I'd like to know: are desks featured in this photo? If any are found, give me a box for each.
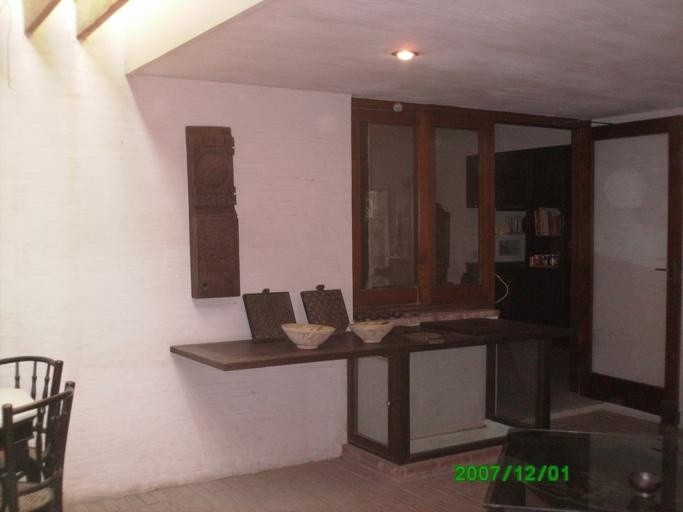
[170,318,571,464]
[484,429,683,512]
[0,388,44,482]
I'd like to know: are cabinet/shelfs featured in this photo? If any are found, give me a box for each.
[467,146,571,346]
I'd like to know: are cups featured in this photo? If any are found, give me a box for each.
[529,254,562,267]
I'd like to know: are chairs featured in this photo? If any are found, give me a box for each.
[0,381,74,512]
[0,357,62,483]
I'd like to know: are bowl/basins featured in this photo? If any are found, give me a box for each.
[348,320,394,344]
[629,470,663,498]
[280,322,336,350]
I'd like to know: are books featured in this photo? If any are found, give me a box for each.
[532,208,565,239]
